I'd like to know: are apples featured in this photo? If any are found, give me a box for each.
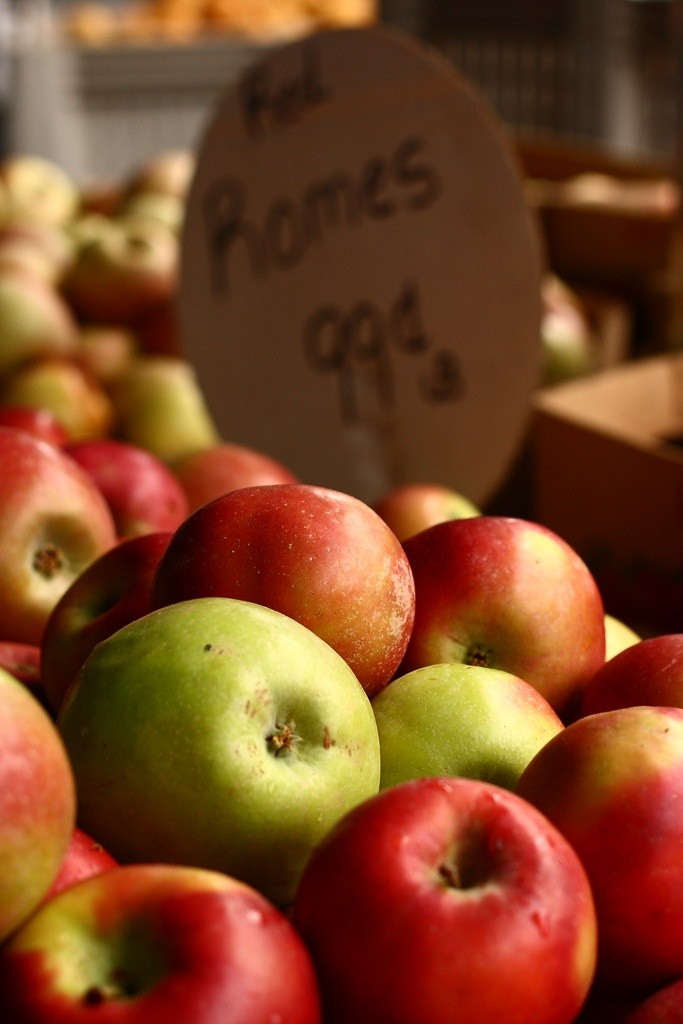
[0,138,683,1024]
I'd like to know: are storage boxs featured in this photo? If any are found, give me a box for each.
[512,121,682,347]
[531,347,683,640]
